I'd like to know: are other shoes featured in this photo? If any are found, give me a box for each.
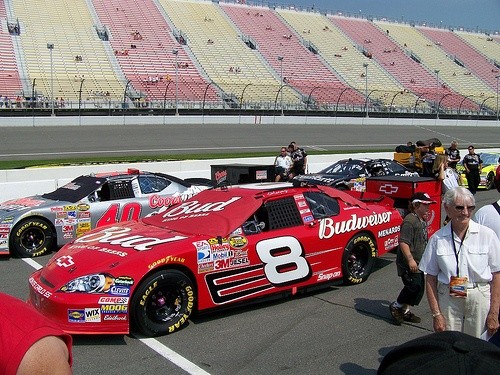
[401,306,417,322]
[389,301,403,324]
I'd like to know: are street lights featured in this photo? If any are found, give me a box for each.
[278,56,284,116]
[362,63,369,118]
[434,69,439,118]
[172,49,180,115]
[47,42,56,115]
[495,74,500,121]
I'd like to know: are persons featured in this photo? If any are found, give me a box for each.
[1,291,76,375]
[59,86,64,92]
[0,0,500,121]
[419,146,439,177]
[418,180,500,342]
[493,157,500,191]
[445,139,461,170]
[274,141,309,183]
[389,191,437,326]
[462,145,484,194]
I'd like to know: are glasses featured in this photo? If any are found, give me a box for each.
[453,204,476,211]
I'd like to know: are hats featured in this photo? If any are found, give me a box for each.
[410,192,437,205]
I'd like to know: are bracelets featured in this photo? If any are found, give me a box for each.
[432,312,442,318]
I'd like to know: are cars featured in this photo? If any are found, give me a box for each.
[27,179,403,339]
[456,152,500,190]
[0,168,213,260]
[288,157,420,189]
